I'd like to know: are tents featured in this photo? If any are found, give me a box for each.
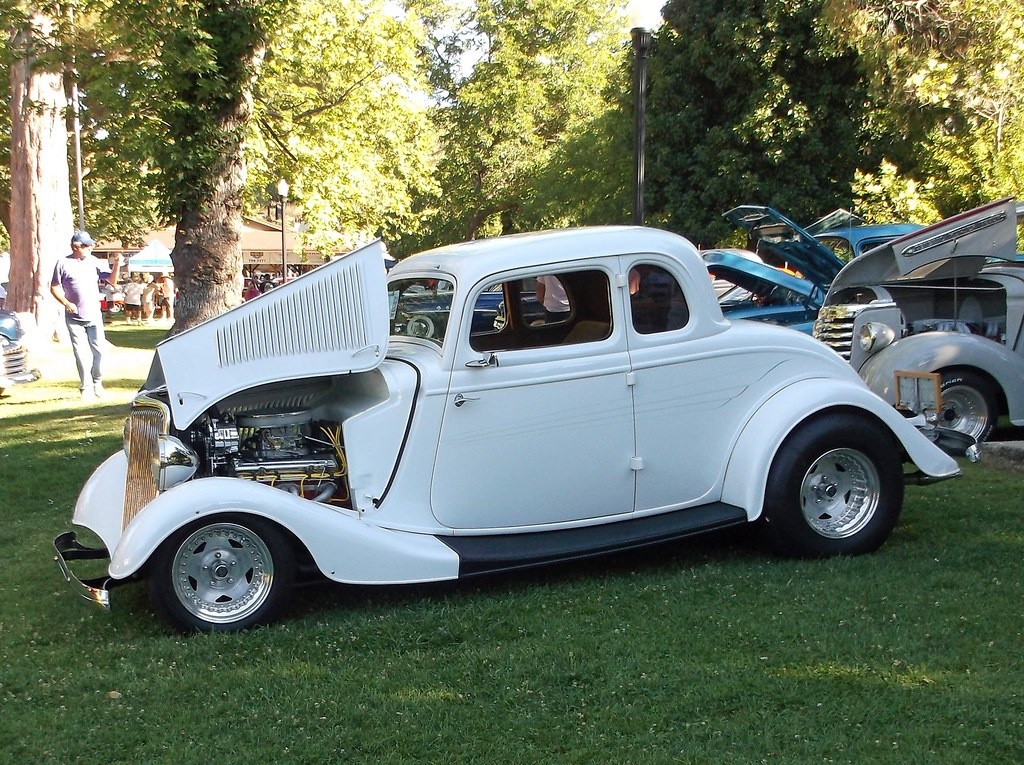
[126,238,177,282]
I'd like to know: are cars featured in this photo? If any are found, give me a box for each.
[50,222,982,635]
[696,194,1024,457]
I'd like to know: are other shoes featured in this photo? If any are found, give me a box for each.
[126,317,175,325]
[80,389,96,402]
[94,380,104,398]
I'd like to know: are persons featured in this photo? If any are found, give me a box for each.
[0,284,7,311]
[536,272,572,326]
[95,268,177,326]
[51,230,125,401]
[237,271,299,303]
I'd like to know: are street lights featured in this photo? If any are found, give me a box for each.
[277,178,291,283]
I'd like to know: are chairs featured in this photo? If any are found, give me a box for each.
[561,318,609,344]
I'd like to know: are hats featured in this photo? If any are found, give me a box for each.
[160,272,172,279]
[70,231,97,246]
